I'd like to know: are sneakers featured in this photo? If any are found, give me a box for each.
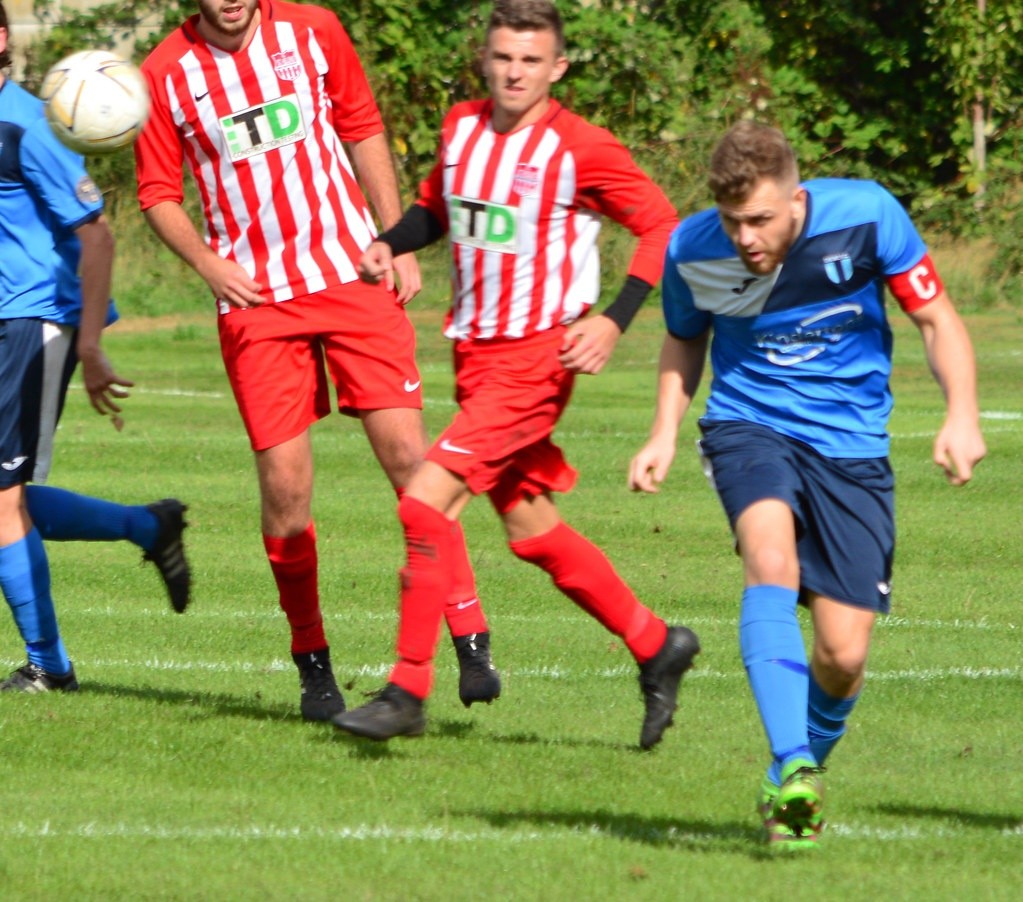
[637,624,700,748]
[0,662,79,695]
[774,757,826,833]
[450,631,500,709]
[140,499,193,613]
[759,777,822,852]
[331,683,426,739]
[292,648,347,721]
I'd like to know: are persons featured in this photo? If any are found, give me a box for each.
[332,0,701,751]
[627,122,987,858]
[133,0,502,727]
[0,0,194,693]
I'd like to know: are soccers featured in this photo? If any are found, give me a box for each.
[40,48,149,155]
